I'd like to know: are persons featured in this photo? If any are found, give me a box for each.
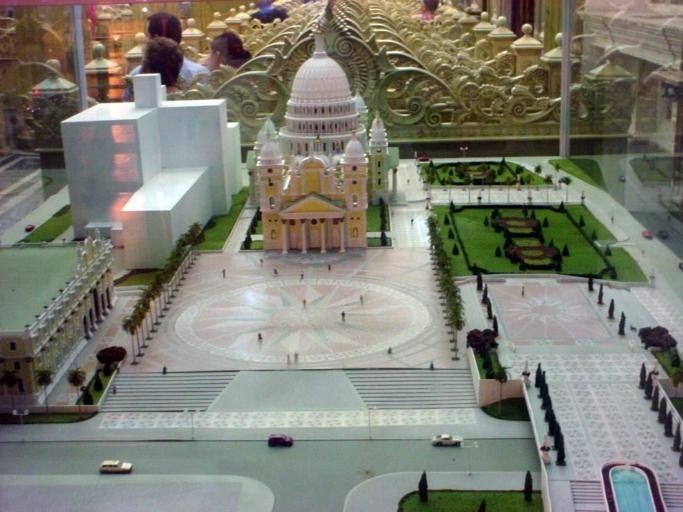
[408,0,439,21]
[126,35,185,102]
[120,10,212,102]
[248,0,288,29]
[206,28,251,71]
